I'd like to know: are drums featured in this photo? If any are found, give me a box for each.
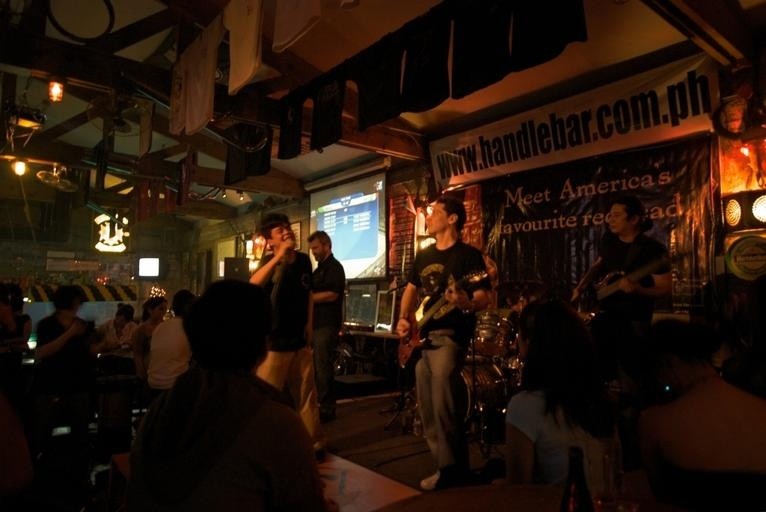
[474,312,512,358]
[501,365,522,391]
[450,362,507,432]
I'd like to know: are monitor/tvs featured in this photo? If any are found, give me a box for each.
[138,257,165,280]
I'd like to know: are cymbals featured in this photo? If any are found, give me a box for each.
[493,282,546,298]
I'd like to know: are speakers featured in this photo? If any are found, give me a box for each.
[224,257,249,282]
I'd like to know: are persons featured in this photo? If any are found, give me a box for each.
[638,326,765,469]
[505,301,585,485]
[397,195,493,491]
[569,198,673,333]
[0,212,348,511]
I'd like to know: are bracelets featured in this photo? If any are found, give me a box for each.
[394,312,410,322]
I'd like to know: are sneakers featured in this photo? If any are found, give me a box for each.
[420,470,440,491]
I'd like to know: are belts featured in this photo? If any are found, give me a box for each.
[429,329,455,337]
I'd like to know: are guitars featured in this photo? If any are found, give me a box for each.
[398,270,488,368]
[570,245,693,320]
